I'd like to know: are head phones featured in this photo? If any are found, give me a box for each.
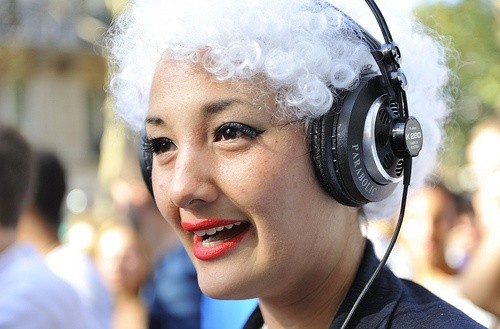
[137,0,422,207]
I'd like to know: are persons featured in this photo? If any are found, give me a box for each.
[0,117,258,329]
[95,0,490,329]
[352,104,499,329]
